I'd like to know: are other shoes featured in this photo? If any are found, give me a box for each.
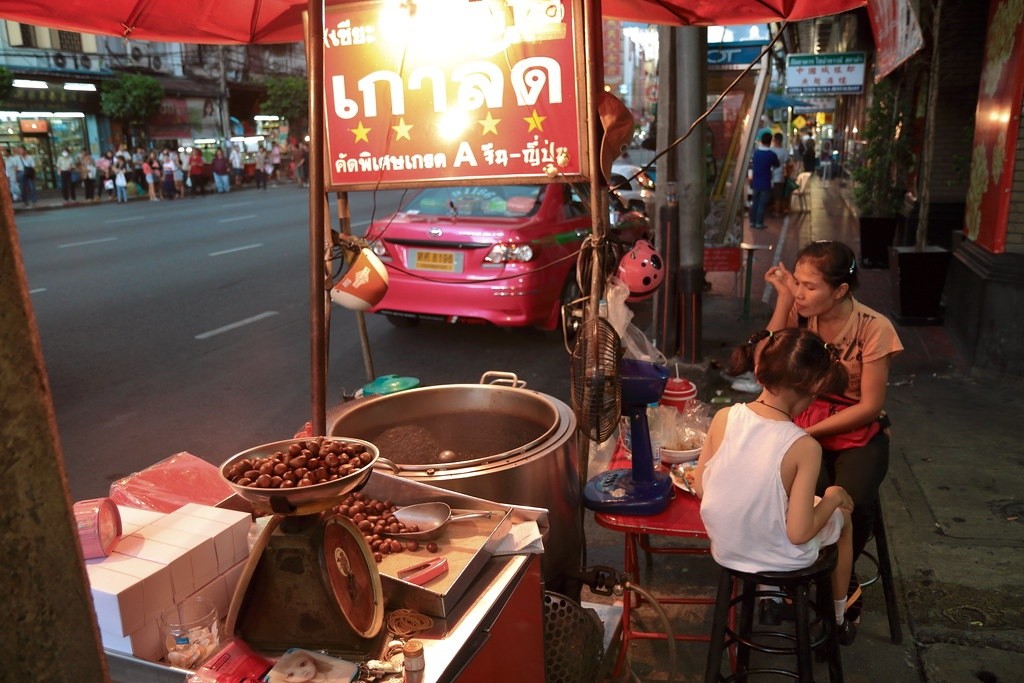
[750,223,768,229]
[830,617,857,644]
[758,599,790,625]
[149,197,160,201]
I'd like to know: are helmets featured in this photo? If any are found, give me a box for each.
[614,240,665,303]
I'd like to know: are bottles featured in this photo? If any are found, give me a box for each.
[646,403,662,468]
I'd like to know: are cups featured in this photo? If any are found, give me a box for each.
[160,597,218,669]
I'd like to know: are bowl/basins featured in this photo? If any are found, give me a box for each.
[651,428,706,463]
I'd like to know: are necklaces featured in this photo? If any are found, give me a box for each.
[755,400,793,421]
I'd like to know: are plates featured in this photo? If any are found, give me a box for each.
[668,461,699,495]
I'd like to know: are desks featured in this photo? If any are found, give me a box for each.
[595,421,746,682]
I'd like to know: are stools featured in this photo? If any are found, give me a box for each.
[845,488,903,645]
[705,547,839,683]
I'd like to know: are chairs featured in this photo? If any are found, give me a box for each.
[787,170,813,215]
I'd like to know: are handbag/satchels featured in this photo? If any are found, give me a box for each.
[104,179,115,190]
[24,166,36,179]
[793,390,890,449]
[186,176,193,187]
[115,169,128,187]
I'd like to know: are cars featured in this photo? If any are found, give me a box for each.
[361,182,654,343]
[533,164,655,227]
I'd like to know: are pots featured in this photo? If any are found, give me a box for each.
[329,369,559,471]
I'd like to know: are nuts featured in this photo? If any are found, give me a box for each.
[330,493,438,562]
[232,435,373,487]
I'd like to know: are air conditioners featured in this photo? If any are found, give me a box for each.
[46,40,172,72]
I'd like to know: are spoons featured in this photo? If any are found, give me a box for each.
[670,464,695,496]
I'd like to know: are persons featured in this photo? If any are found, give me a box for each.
[770,131,816,218]
[754,239,905,624]
[750,132,780,229]
[3,138,310,208]
[199,99,220,140]
[695,327,869,647]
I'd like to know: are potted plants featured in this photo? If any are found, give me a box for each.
[884,1,965,327]
[852,80,911,270]
